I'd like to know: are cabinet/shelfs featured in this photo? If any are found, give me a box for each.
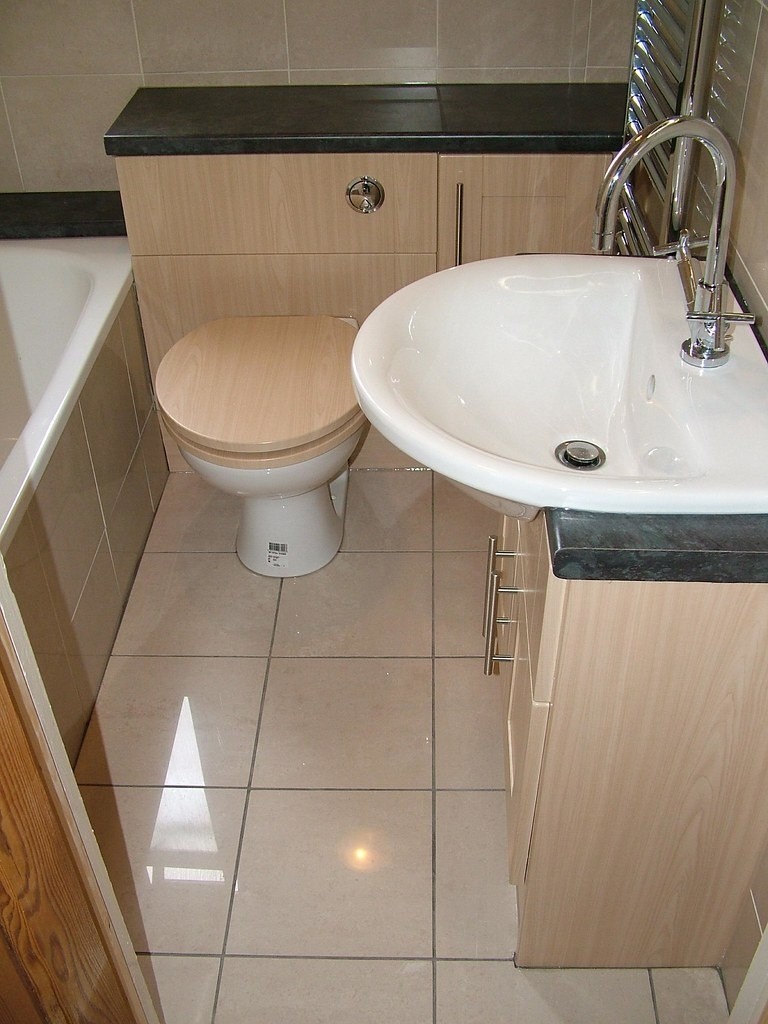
[100,79,675,474]
[482,505,768,972]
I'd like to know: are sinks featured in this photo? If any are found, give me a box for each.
[349,253,768,516]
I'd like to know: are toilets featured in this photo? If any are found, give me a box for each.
[154,314,369,579]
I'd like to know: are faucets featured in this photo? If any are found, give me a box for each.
[591,115,756,369]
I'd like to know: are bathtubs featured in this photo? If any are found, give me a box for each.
[0,236,171,774]
